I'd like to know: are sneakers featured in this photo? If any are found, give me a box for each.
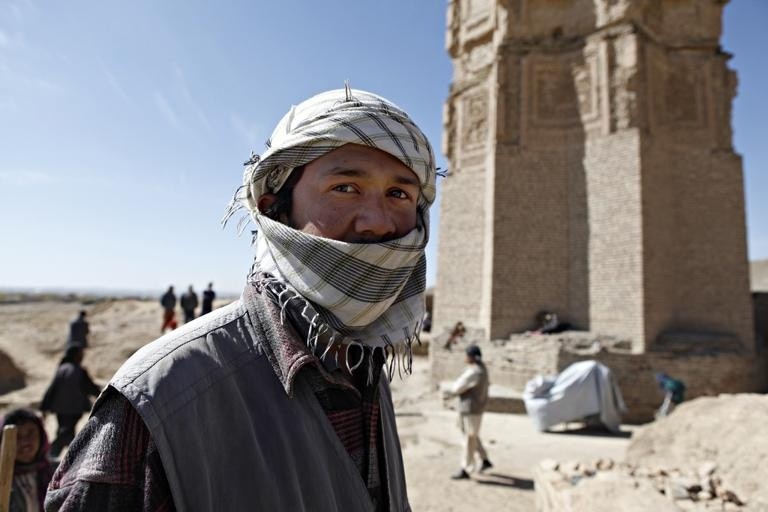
[449,460,492,481]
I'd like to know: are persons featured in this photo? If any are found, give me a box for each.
[42,87,439,512]
[160,285,178,334]
[655,373,686,416]
[436,345,494,481]
[181,284,199,324]
[0,407,54,512]
[200,282,216,316]
[67,309,90,350]
[38,342,102,458]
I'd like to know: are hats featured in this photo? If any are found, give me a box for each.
[466,345,481,358]
